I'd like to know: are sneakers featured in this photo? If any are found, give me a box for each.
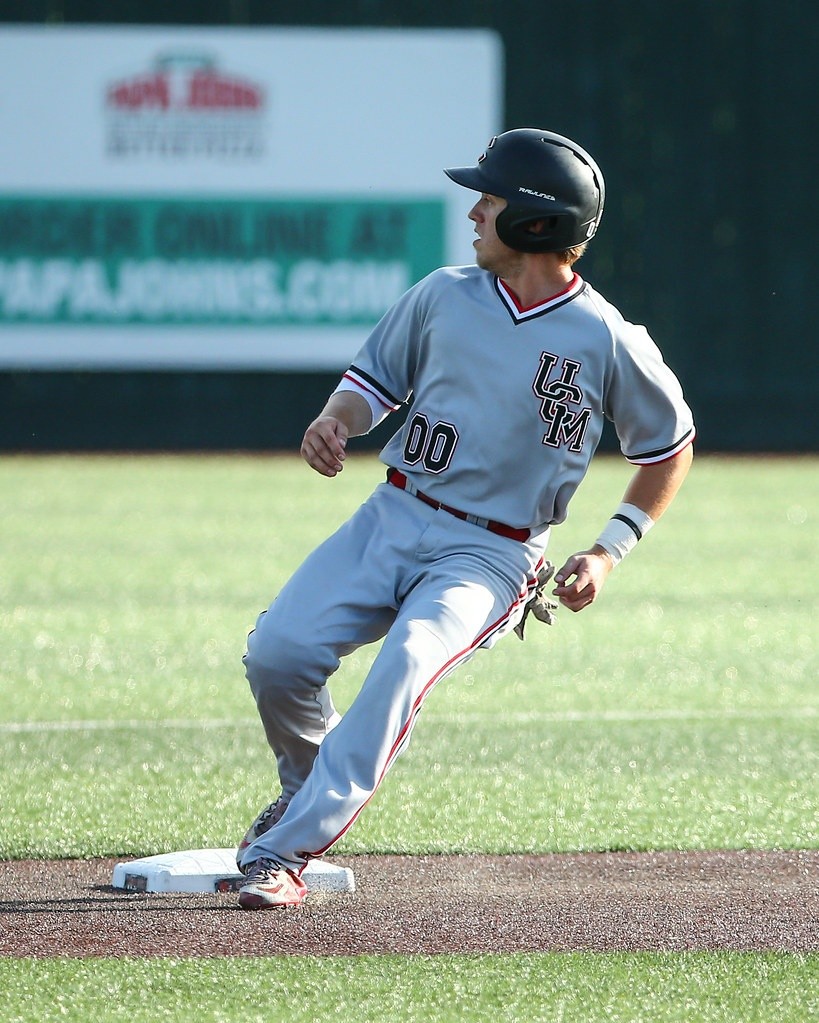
[237,855,307,909]
[235,794,292,873]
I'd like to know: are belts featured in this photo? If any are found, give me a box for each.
[387,469,530,544]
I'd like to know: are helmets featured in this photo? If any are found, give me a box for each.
[443,128,606,253]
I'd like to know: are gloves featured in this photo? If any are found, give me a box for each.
[513,559,559,641]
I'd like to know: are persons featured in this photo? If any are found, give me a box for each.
[236,129,696,910]
